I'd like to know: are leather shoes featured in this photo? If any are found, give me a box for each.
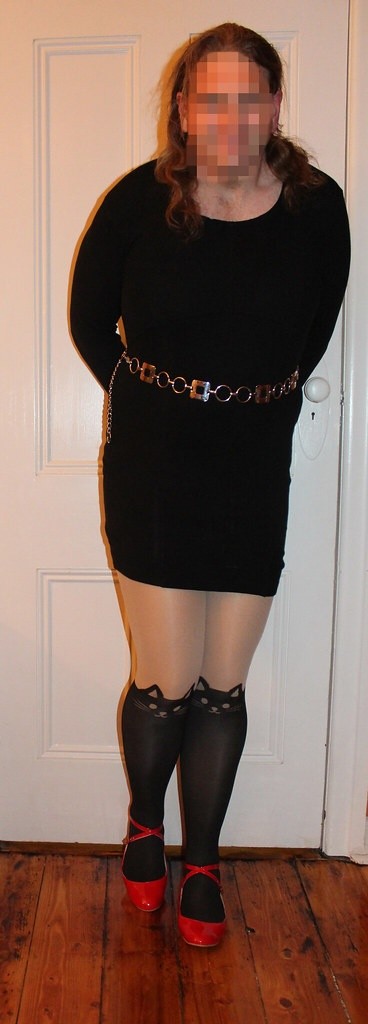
[177,860,228,947]
[121,806,169,912]
